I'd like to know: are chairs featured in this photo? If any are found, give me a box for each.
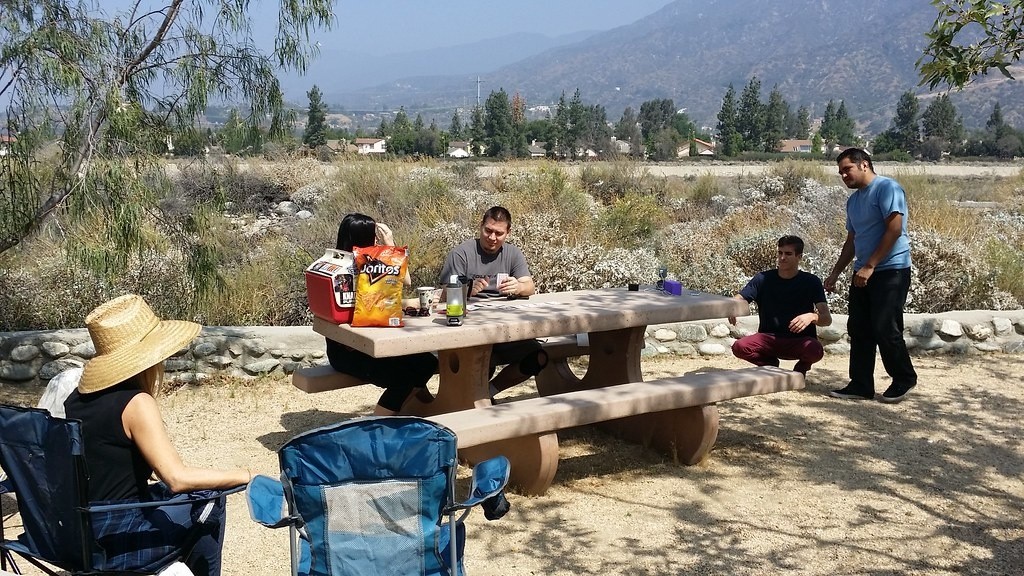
[246,415,508,576]
[0,403,249,576]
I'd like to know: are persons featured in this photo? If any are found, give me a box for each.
[325,214,440,418]
[63,294,263,576]
[732,236,833,380]
[825,148,917,402]
[440,208,550,407]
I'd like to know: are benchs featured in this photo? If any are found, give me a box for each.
[291,339,805,495]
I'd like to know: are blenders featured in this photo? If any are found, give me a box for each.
[444,283,464,325]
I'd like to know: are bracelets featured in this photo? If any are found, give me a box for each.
[246,468,252,481]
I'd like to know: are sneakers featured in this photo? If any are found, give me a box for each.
[882,379,917,402]
[830,383,865,399]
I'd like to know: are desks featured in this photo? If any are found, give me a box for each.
[314,286,748,495]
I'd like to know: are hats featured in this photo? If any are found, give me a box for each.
[78,294,202,394]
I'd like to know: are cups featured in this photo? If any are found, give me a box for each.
[416,287,434,316]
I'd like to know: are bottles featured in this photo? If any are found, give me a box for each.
[455,275,473,316]
